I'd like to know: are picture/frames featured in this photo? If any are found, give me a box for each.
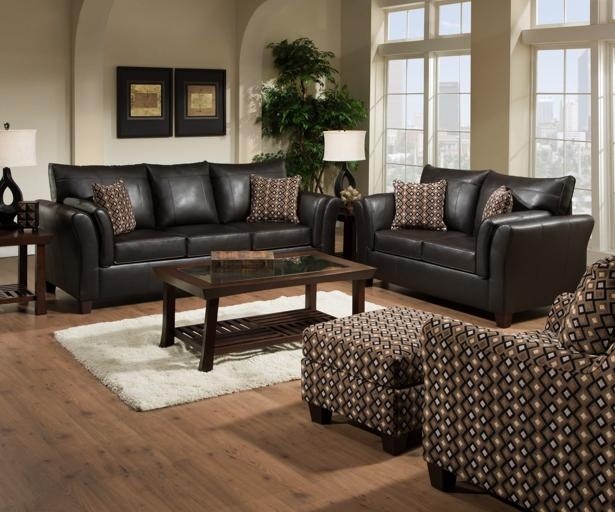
[174,67,227,137]
[116,64,173,138]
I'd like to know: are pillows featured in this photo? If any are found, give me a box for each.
[558,259,614,352]
[473,168,576,230]
[89,178,138,235]
[147,161,219,228]
[209,158,286,224]
[481,184,513,220]
[422,163,487,234]
[49,162,156,232]
[248,172,302,226]
[390,177,447,232]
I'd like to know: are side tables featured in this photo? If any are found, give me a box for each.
[1,227,52,315]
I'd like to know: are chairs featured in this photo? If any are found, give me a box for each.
[421,255,615,511]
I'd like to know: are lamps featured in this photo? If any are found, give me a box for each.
[1,120,37,232]
[322,128,367,197]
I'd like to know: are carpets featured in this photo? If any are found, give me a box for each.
[51,289,384,413]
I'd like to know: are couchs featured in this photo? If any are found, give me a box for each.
[356,164,597,327]
[34,160,340,316]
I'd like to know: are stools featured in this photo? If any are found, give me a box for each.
[301,303,479,456]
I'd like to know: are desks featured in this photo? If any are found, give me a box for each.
[337,213,357,258]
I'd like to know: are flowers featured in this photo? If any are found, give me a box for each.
[339,185,360,204]
